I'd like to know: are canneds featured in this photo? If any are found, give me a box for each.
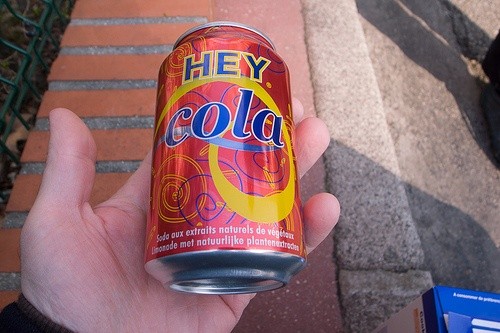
[144,19,306,296]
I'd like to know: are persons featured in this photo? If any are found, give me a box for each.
[1,97,339,332]
